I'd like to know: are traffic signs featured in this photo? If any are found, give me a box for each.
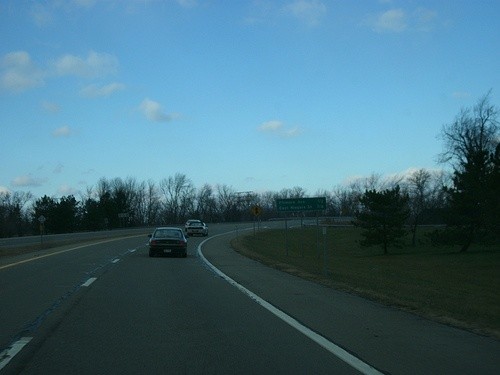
[276,197,327,212]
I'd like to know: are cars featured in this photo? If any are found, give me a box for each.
[147,227,188,258]
[185,219,208,237]
